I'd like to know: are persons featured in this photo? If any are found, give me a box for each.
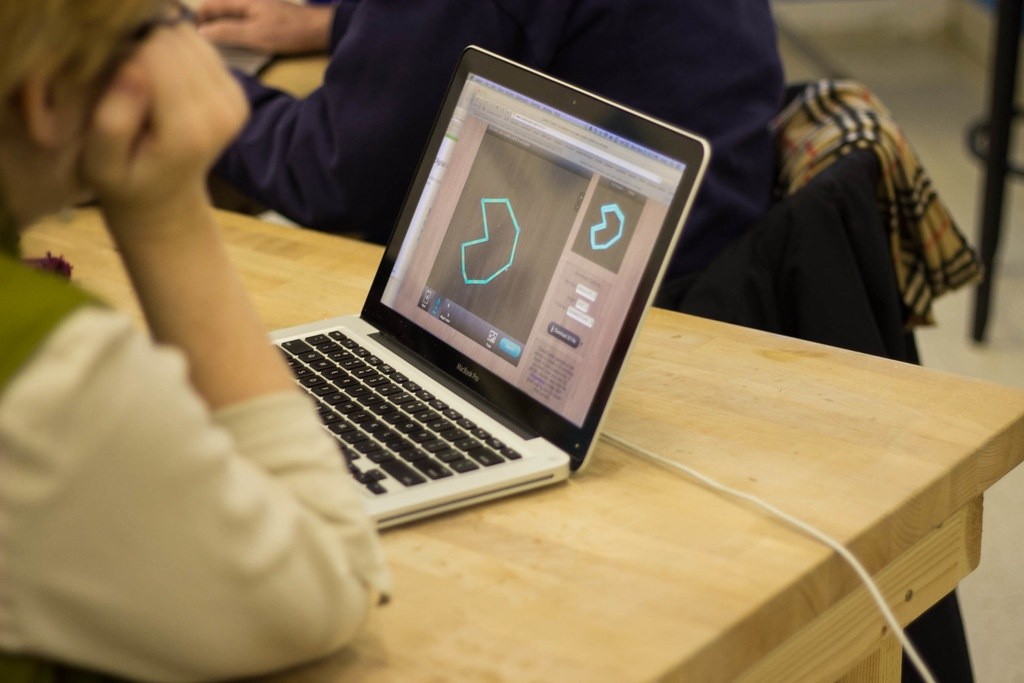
[0,0,393,683]
[196,0,784,313]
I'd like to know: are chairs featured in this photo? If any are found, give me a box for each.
[677,80,979,682]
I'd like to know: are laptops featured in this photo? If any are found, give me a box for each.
[265,43,711,528]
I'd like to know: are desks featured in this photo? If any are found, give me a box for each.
[25,206,1024,682]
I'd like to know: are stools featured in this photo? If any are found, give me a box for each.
[964,1,1023,341]
